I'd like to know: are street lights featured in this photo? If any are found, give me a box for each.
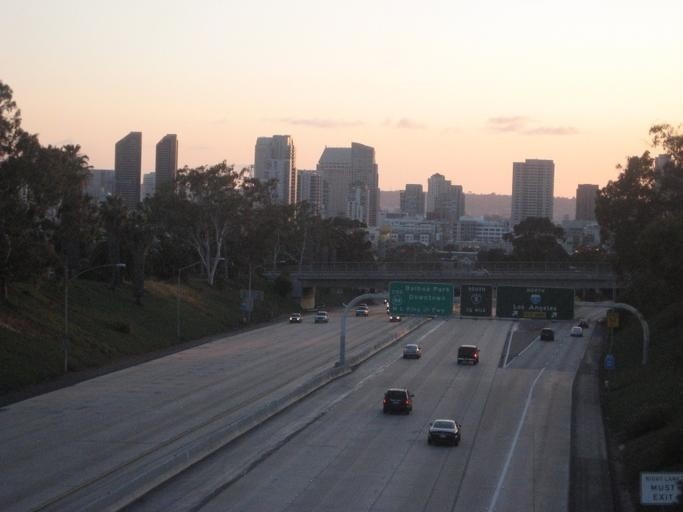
[176,257,225,346]
[64,264,127,375]
[247,260,287,324]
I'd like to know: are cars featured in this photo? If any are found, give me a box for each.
[356,303,369,316]
[403,343,423,359]
[571,319,589,337]
[383,388,415,414]
[289,312,303,323]
[390,315,401,322]
[541,328,555,341]
[428,419,462,446]
[314,311,330,323]
[457,345,481,365]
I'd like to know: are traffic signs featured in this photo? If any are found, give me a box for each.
[496,285,574,319]
